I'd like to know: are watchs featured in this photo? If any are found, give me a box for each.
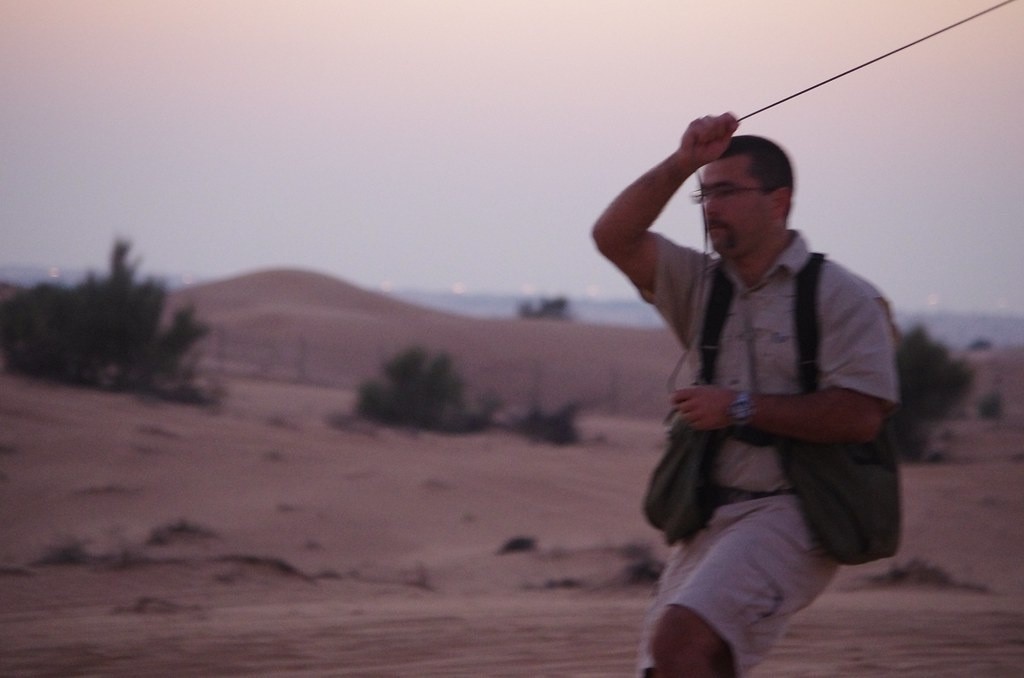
[729,390,755,426]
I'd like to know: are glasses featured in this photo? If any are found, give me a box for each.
[689,183,773,206]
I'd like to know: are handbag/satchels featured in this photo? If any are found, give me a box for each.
[781,430,900,566]
[640,407,726,545]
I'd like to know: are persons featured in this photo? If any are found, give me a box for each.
[593,113,904,678]
